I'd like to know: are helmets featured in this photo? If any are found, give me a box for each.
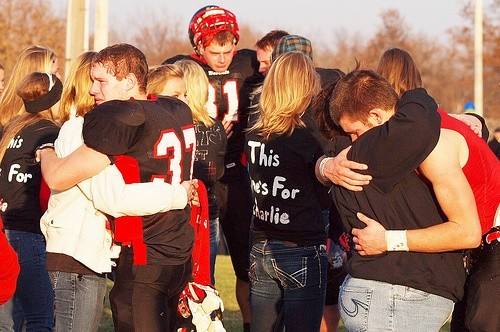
[188,4,240,56]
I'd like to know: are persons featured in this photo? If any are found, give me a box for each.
[315,69,500,332]
[0,0,500,332]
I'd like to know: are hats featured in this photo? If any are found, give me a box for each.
[271,34,313,63]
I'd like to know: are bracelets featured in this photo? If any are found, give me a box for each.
[386,230,408,251]
[319,157,333,182]
